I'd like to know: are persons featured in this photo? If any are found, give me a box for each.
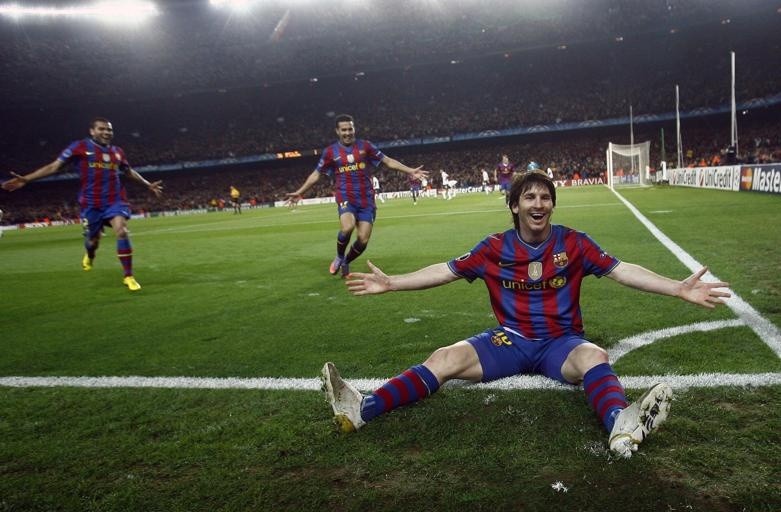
[0,1,781,230]
[1,116,167,293]
[283,114,431,281]
[320,167,736,461]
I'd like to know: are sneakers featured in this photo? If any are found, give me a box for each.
[83,251,94,271]
[341,256,350,277]
[607,381,680,461]
[329,255,343,275]
[321,359,368,436]
[123,275,142,291]
